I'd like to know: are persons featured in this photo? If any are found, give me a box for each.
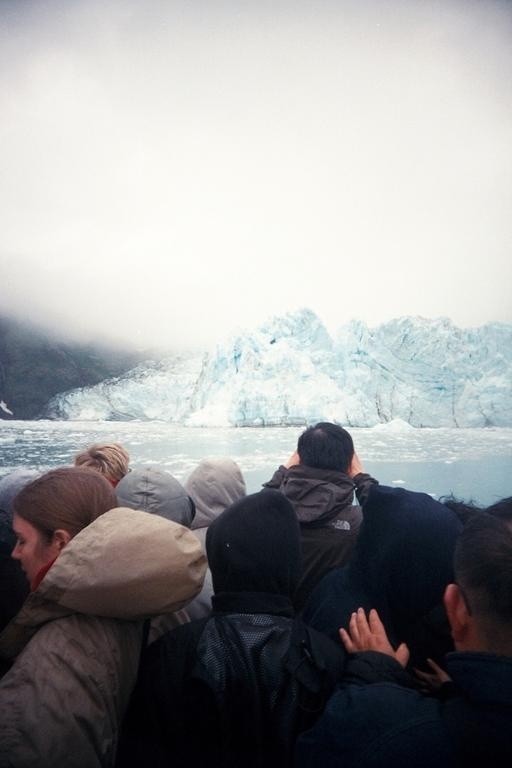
[1,421,512,767]
